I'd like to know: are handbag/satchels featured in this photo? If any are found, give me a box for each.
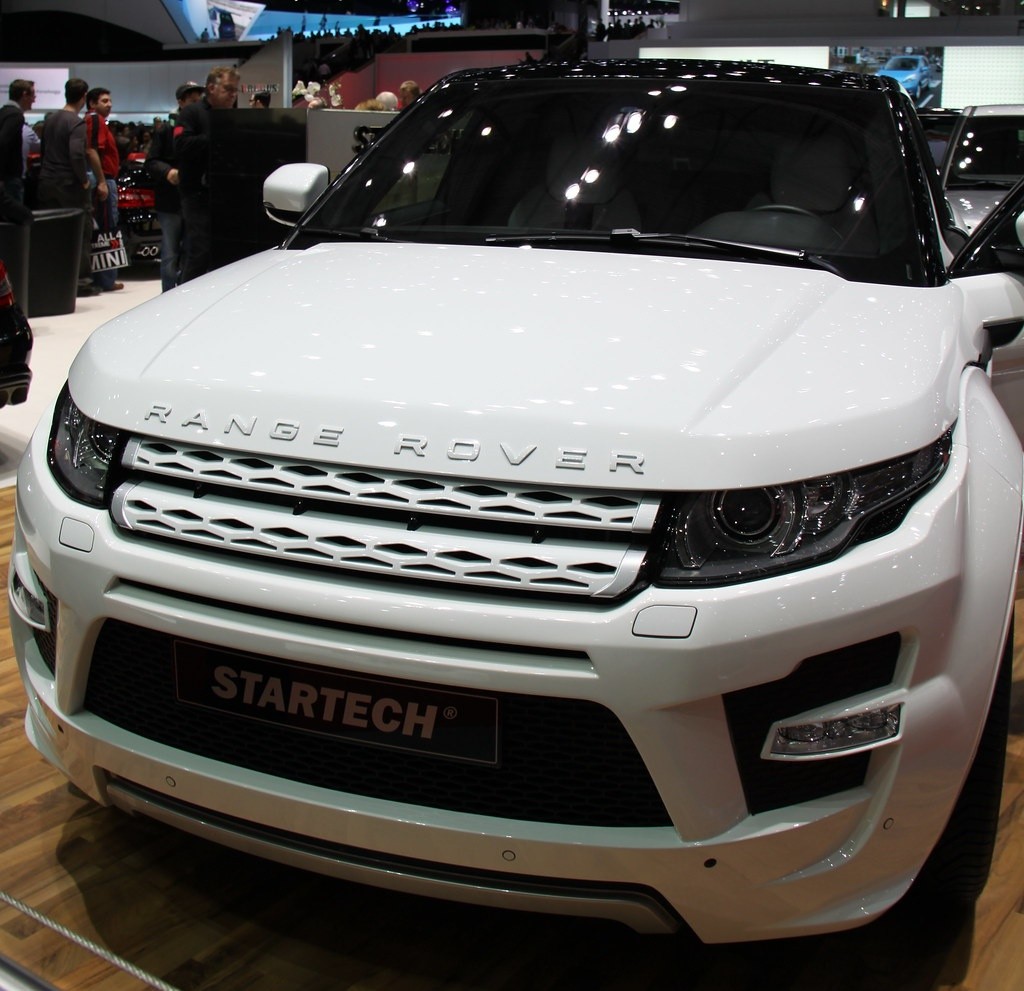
[91,227,132,271]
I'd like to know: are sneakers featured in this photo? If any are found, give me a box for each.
[102,283,124,291]
[77,286,101,296]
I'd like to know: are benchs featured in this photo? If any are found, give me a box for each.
[605,108,830,236]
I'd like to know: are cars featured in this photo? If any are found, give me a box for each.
[112,154,167,262]
[10,64,1023,977]
[876,55,932,101]
[936,105,1024,253]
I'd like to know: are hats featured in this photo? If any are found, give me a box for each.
[176,85,205,98]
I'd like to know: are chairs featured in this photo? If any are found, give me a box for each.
[743,132,881,254]
[970,120,1024,174]
[508,129,644,232]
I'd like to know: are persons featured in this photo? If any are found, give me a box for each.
[0,80,53,210]
[39,77,93,273]
[82,88,123,292]
[354,90,398,111]
[399,80,421,110]
[143,81,207,294]
[249,90,272,109]
[107,117,166,170]
[172,69,240,285]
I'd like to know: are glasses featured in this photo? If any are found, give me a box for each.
[249,99,255,102]
